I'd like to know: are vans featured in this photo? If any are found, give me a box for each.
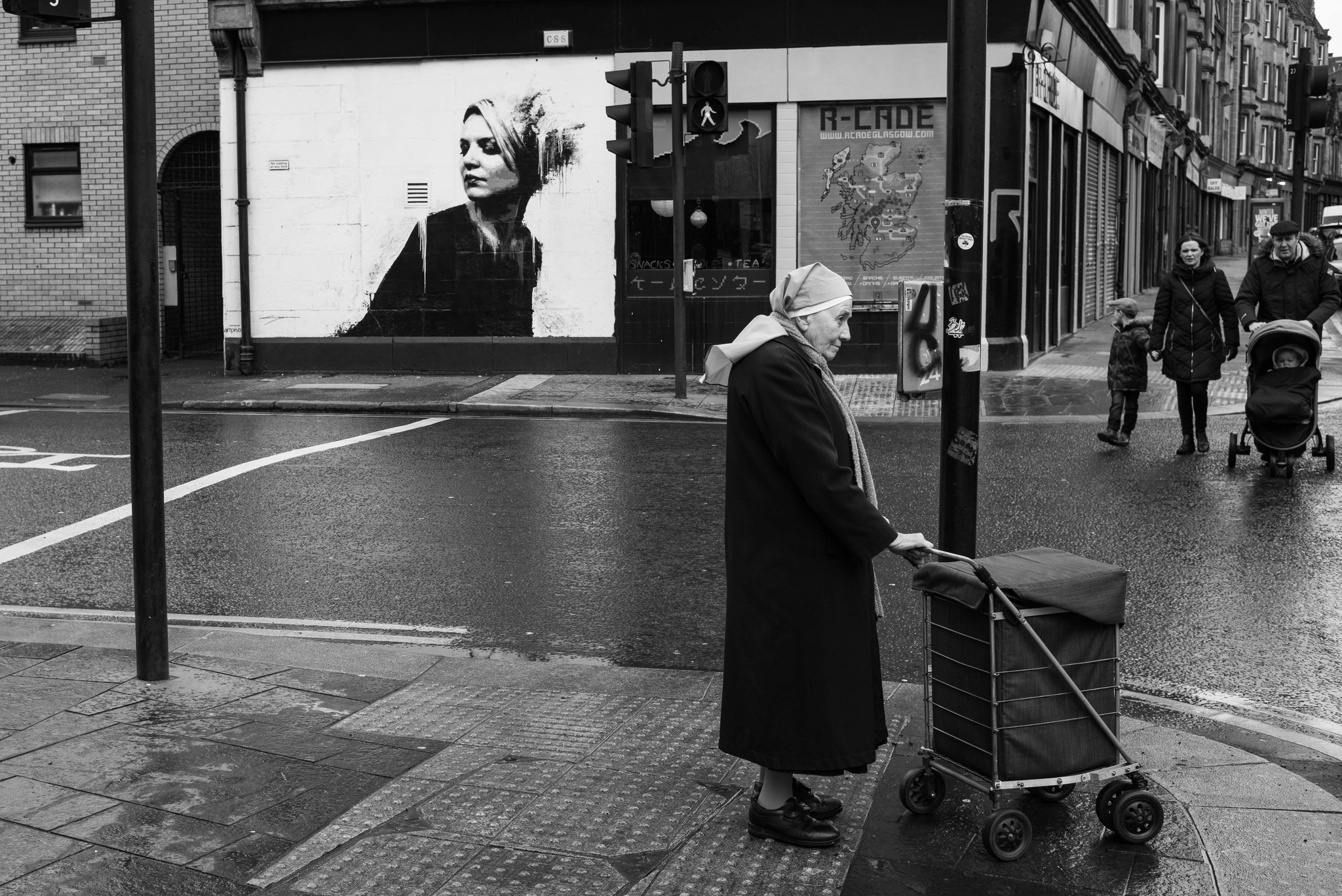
[1322,205,1342,225]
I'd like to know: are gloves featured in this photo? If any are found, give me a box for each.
[1150,348,1164,362]
[1225,344,1238,361]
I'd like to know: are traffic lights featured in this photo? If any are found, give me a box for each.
[1285,63,1331,132]
[2,0,80,23]
[605,61,655,169]
[686,60,729,135]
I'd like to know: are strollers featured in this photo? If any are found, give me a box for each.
[1227,319,1335,479]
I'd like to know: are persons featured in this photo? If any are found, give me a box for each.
[699,262,934,848]
[1149,232,1240,454]
[1234,221,1342,462]
[1272,343,1308,369]
[349,95,573,336]
[1097,297,1161,446]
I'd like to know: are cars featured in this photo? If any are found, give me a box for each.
[1320,222,1342,291]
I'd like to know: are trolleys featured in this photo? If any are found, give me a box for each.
[898,540,1166,864]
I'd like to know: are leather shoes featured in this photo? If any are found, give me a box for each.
[751,778,842,821]
[748,793,841,848]
[1097,428,1119,446]
[1195,431,1209,453]
[1176,433,1196,455]
[1111,433,1131,447]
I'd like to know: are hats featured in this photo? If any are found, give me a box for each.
[1109,297,1139,315]
[1269,220,1300,236]
